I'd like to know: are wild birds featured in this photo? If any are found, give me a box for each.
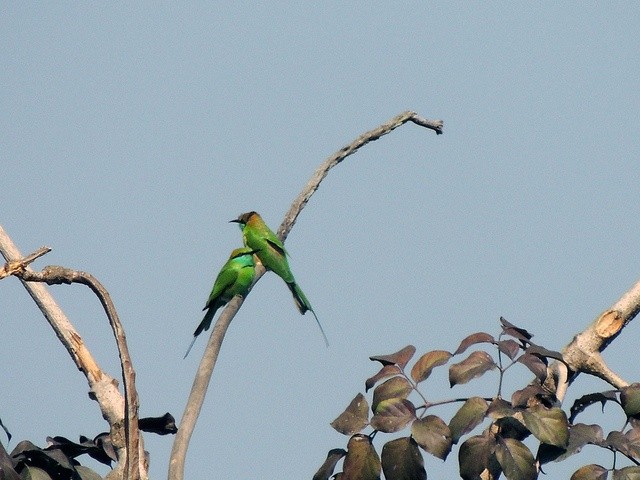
[181,245,260,360]
[230,210,330,346]
